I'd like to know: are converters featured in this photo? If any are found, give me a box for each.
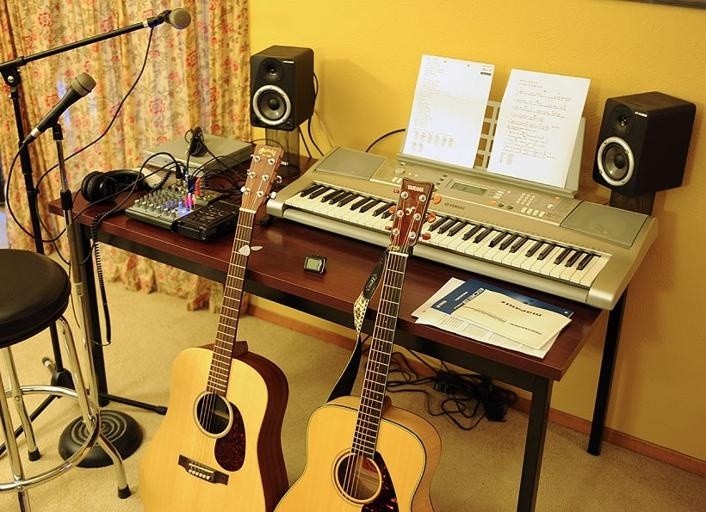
[434,370,462,395]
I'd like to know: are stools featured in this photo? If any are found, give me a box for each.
[0,250,131,512]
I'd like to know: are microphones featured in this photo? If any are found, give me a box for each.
[22,72,96,146]
[164,8,191,29]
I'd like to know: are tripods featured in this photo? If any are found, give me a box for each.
[0,11,167,456]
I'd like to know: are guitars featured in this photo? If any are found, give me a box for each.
[274,178,442,512]
[138,143,289,511]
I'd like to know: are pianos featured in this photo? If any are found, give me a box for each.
[265,147,659,312]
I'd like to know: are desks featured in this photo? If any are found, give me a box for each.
[51,155,647,510]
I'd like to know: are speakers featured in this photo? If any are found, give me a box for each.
[250,46,313,131]
[592,92,696,197]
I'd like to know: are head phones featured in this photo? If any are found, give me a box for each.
[81,170,144,203]
